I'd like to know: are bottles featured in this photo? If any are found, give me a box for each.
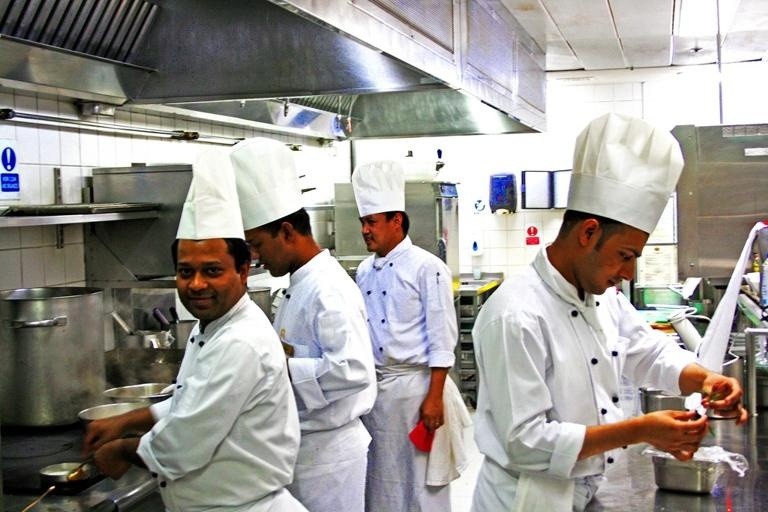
[753,253,768,307]
[169,305,180,322]
[107,309,134,336]
[152,307,171,333]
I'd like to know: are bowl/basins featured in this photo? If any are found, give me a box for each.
[105,382,172,402]
[79,400,153,424]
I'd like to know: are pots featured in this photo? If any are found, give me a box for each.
[38,450,104,490]
[1,282,105,432]
[638,386,685,415]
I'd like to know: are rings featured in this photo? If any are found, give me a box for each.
[435,422,440,425]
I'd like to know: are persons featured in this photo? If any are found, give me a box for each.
[469,209,750,512]
[355,209,459,512]
[243,208,378,511]
[80,238,311,511]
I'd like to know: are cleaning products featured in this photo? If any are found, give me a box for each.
[471,254,482,280]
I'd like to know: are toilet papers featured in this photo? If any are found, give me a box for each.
[669,311,702,351]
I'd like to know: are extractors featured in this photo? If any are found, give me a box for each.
[0,1,168,74]
[272,95,361,120]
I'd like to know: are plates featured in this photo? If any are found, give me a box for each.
[731,332,759,347]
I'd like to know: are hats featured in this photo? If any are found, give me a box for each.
[175,155,247,241]
[567,112,685,234]
[351,159,405,218]
[230,137,303,231]
